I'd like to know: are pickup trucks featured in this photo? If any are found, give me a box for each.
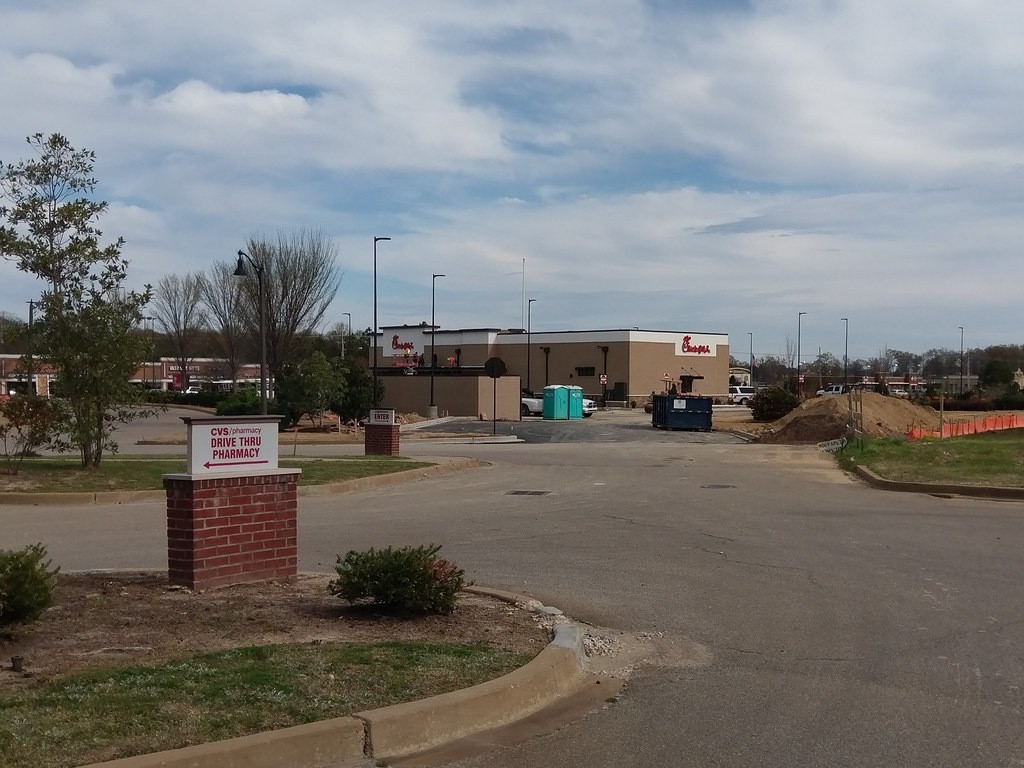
[521,397,598,418]
[816,385,845,397]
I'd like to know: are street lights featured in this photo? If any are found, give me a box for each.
[798,312,806,398]
[373,236,391,408]
[959,327,964,394]
[343,313,351,335]
[141,316,156,389]
[748,333,753,385]
[231,249,268,415]
[840,318,847,391]
[528,298,537,393]
[431,274,445,405]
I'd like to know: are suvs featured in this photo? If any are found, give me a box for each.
[890,390,908,395]
[729,386,756,405]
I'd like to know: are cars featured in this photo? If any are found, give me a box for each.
[256,386,274,398]
[182,386,202,393]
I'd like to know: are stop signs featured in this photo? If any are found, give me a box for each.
[600,375,607,384]
[665,373,669,380]
